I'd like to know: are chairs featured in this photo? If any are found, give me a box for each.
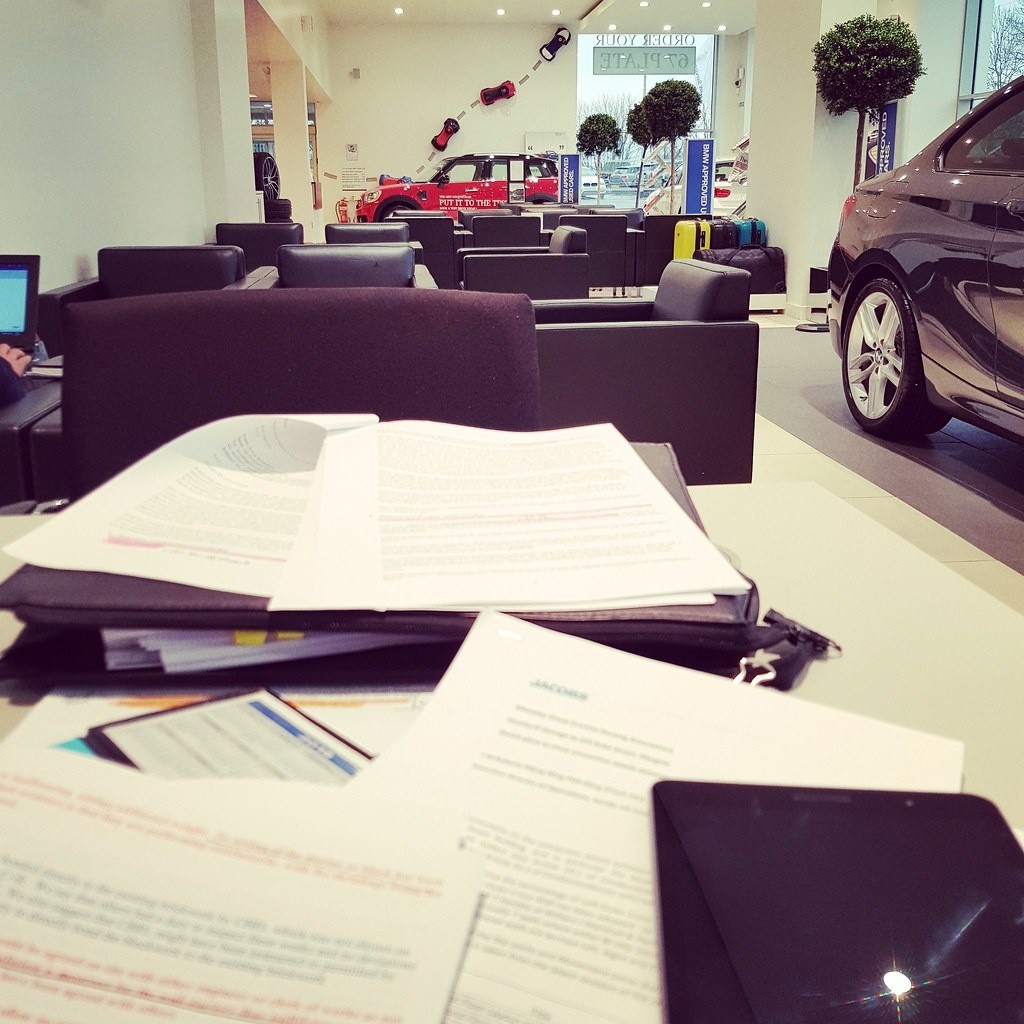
[0,202,759,486]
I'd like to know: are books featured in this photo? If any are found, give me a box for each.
[25,352,64,380]
[55,690,378,794]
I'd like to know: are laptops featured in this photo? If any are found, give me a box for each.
[0,255,41,372]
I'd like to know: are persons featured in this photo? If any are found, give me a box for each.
[0,406,763,690]
[0,342,32,408]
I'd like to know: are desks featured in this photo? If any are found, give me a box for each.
[1,482,1023,846]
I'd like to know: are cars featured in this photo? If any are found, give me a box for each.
[642,158,747,219]
[597,158,684,197]
[478,80,517,107]
[581,165,607,199]
[538,28,572,62]
[430,117,461,154]
[827,72,1023,455]
[355,150,560,223]
